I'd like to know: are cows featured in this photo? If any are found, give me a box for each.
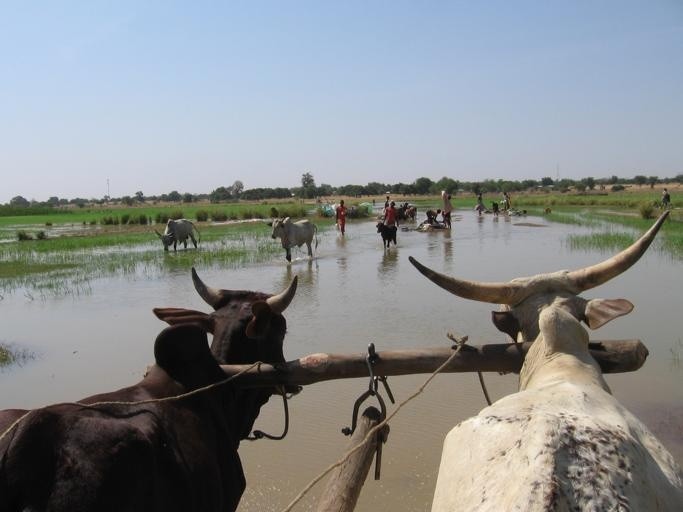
[0,265,299,512]
[153,217,201,252]
[375,221,399,249]
[406,209,683,512]
[267,215,319,263]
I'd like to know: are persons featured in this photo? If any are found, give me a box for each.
[499,192,509,211]
[384,196,389,209]
[491,202,498,216]
[473,192,487,216]
[660,188,670,207]
[425,208,440,225]
[441,190,453,229]
[334,200,347,238]
[383,201,399,227]
